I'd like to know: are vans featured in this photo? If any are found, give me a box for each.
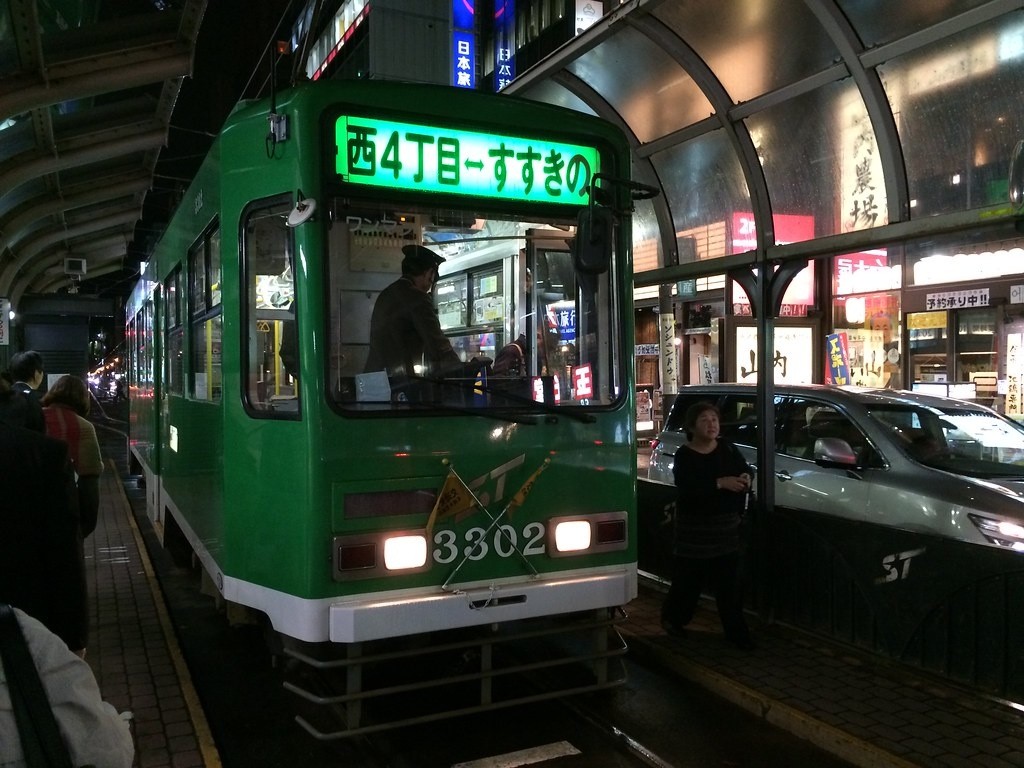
[648,383,1024,554]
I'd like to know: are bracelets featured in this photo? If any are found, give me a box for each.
[717,479,720,489]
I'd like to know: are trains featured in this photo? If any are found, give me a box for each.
[127,78,659,740]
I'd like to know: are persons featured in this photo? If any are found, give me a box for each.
[1,351,105,659]
[362,244,461,374]
[911,434,950,472]
[280,300,299,381]
[116,380,128,401]
[0,601,135,768]
[493,321,560,378]
[659,401,755,648]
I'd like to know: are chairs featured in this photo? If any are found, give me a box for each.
[800,411,872,468]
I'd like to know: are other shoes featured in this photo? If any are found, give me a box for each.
[723,627,763,644]
[660,616,686,635]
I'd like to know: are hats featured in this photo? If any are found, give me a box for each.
[402,245,447,268]
